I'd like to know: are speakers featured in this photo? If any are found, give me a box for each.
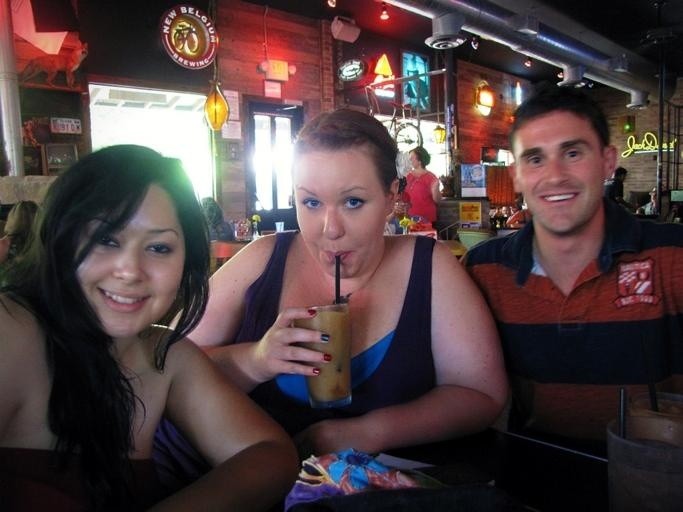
[332,20,361,44]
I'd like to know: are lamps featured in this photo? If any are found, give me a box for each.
[434,50,446,143]
[202,0,229,131]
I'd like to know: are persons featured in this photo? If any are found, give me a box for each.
[200,196,233,245]
[454,86,682,446]
[1,143,301,511]
[150,106,512,512]
[402,147,442,229]
[405,70,427,100]
[602,166,639,213]
[385,191,428,234]
[634,184,659,215]
[0,199,42,265]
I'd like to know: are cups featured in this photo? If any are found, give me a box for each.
[293,304,353,409]
[275,221,284,236]
[605,392,683,511]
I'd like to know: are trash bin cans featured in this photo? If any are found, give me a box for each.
[456,229,492,250]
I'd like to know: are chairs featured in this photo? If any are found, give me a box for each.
[209,241,247,276]
